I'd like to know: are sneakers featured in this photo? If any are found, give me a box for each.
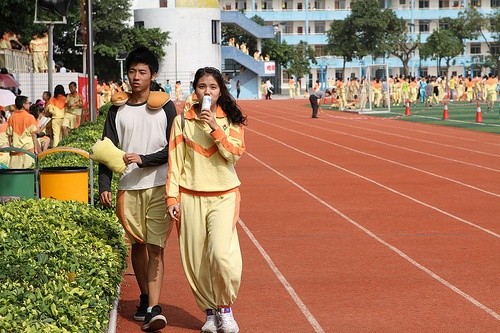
[201,306,218,333]
[141,304,167,333]
[215,307,239,333]
[133,293,149,321]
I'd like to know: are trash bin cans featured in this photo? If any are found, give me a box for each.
[0,146,38,204]
[37,145,94,209]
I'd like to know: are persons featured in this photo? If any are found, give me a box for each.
[289,76,301,100]
[261,79,274,99]
[98,47,177,331]
[97,79,128,110]
[0,67,83,169]
[159,79,194,101]
[254,50,270,61]
[225,65,246,98]
[309,91,331,118]
[304,73,500,111]
[165,67,248,333]
[229,37,249,55]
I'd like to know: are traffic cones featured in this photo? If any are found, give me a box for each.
[442,102,449,120]
[404,100,410,116]
[475,102,483,123]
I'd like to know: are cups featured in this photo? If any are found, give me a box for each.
[201,95,212,113]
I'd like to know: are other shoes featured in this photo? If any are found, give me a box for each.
[392,103,399,107]
[312,116,318,118]
[487,106,494,112]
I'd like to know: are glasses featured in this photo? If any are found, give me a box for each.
[25,102,30,105]
